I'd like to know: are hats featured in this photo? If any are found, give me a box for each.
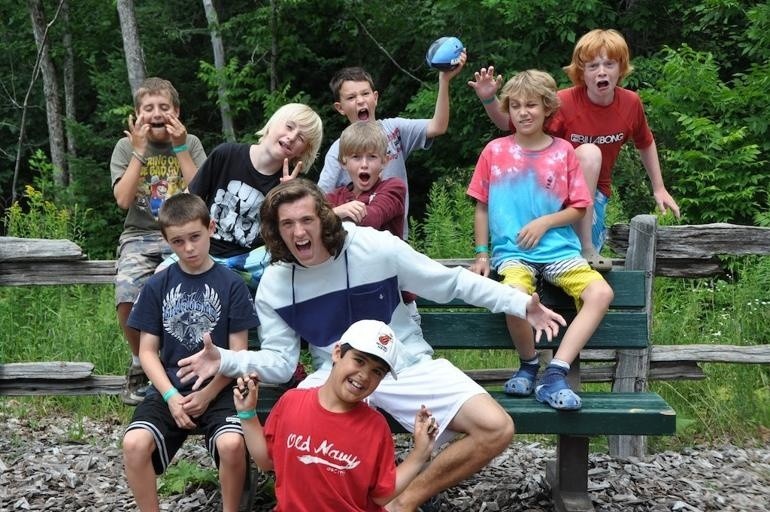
[339,319,401,381]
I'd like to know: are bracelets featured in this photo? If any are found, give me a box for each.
[474,245,489,254]
[481,94,496,105]
[162,387,178,402]
[131,150,148,166]
[171,144,188,153]
[236,409,257,420]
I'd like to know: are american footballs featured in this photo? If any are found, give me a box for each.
[426,36,463,70]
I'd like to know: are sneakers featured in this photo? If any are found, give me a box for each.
[120,363,150,407]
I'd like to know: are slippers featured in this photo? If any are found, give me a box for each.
[588,247,614,271]
[504,370,539,399]
[535,380,585,411]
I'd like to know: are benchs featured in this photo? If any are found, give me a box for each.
[160,267,675,512]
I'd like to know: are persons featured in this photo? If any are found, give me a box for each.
[175,177,568,512]
[316,47,467,241]
[467,27,680,271]
[130,102,324,402]
[323,119,422,329]
[465,69,615,410]
[122,191,260,512]
[109,77,208,405]
[231,318,440,512]
